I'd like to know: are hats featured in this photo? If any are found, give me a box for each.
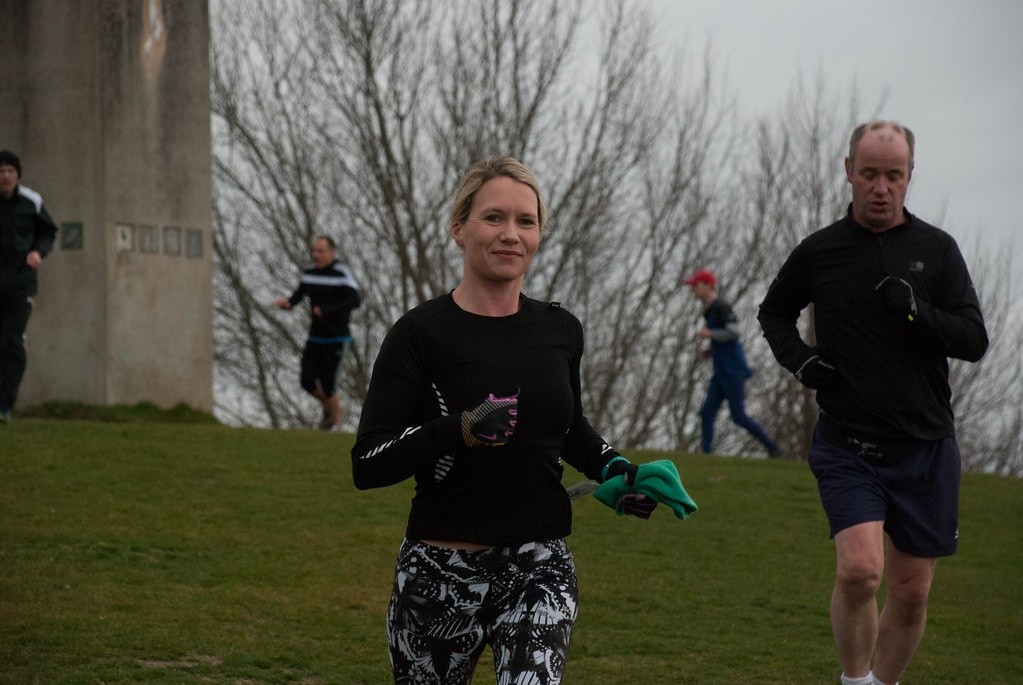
[679,271,716,289]
[0,150,21,178]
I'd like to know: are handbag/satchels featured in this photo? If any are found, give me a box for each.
[818,414,925,467]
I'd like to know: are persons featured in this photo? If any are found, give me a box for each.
[756,121,989,685]
[352,156,659,685]
[0,148,60,424]
[683,269,786,459]
[276,235,362,430]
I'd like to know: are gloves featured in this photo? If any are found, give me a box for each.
[423,386,520,455]
[874,273,921,331]
[600,455,660,520]
[793,348,844,390]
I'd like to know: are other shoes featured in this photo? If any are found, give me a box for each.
[317,414,337,430]
[0,408,10,423]
[767,449,784,459]
